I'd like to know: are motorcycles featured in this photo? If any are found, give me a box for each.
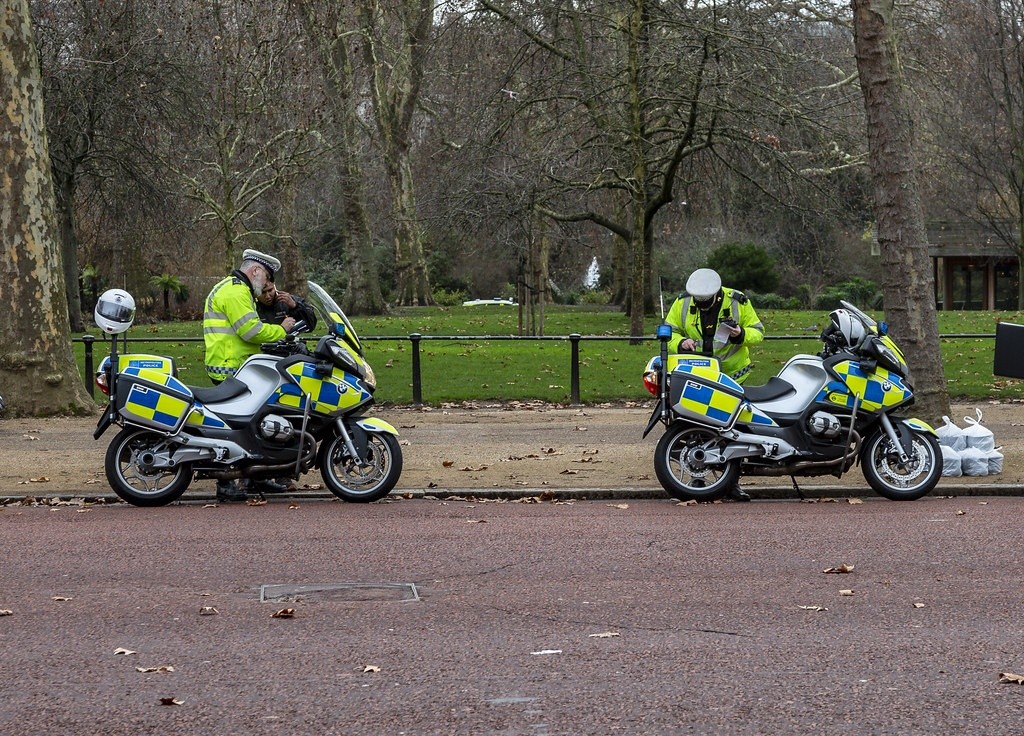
[93,282,402,507]
[644,298,943,502]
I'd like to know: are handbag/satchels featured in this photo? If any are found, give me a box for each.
[935,408,1005,477]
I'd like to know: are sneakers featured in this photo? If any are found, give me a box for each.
[247,479,287,492]
[724,483,751,501]
[275,477,298,491]
[216,483,264,503]
[692,480,706,488]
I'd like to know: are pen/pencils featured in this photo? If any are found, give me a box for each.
[694,339,698,343]
[283,312,287,319]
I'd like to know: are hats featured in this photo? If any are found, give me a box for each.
[686,268,722,309]
[242,249,281,283]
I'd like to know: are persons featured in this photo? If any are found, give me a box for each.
[204,250,296,501]
[664,268,765,502]
[256,277,318,489]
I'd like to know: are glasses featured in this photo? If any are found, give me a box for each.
[255,266,270,279]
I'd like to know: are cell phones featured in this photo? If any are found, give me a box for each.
[273,283,279,296]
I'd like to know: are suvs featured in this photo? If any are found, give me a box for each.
[462,297,518,306]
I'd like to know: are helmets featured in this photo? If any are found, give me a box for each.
[94,289,137,335]
[823,309,866,352]
